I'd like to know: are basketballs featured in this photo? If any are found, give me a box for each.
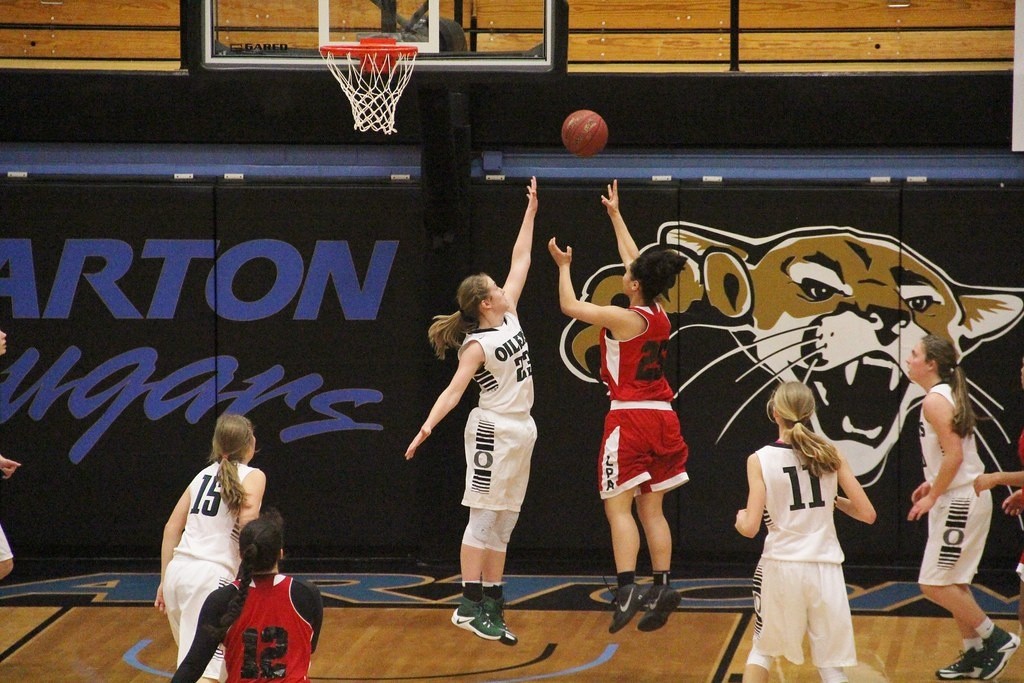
[559,110,609,158]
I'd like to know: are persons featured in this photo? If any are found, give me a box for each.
[972,352,1024,628]
[171,507,323,683]
[735,382,876,683]
[907,335,1021,682]
[548,180,691,635]
[0,330,21,580]
[404,174,539,645]
[154,414,267,683]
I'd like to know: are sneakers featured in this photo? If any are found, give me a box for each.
[979,624,1021,680]
[638,584,681,632]
[450,597,505,641]
[936,647,983,680]
[604,576,648,634]
[483,594,517,645]
